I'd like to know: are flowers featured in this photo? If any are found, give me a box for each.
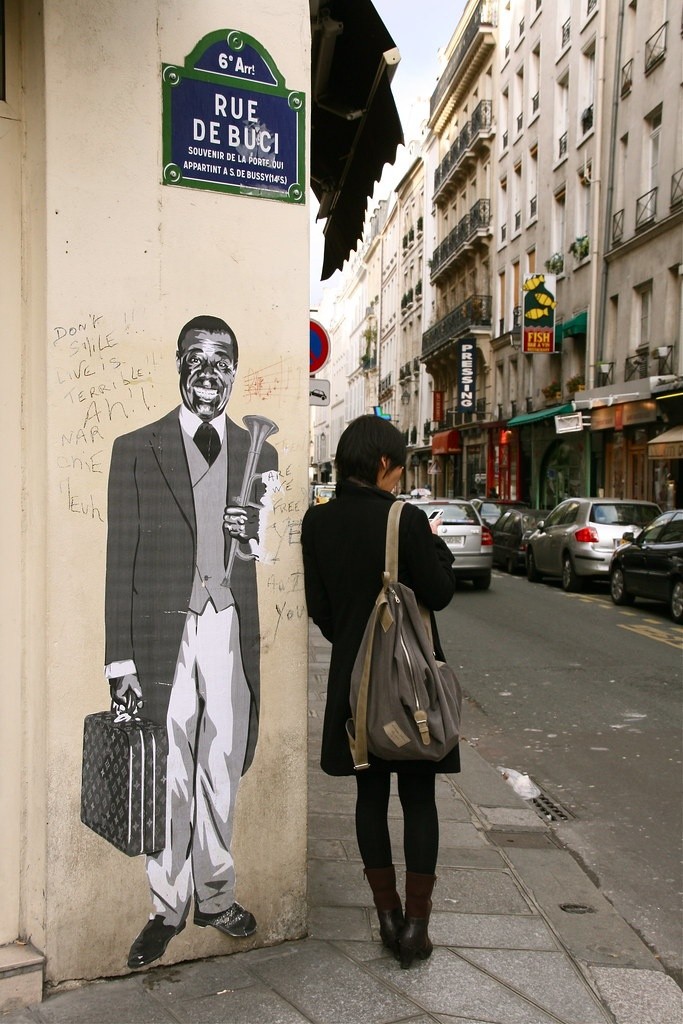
[542,379,560,398]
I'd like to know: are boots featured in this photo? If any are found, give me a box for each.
[363,864,405,961]
[399,870,432,968]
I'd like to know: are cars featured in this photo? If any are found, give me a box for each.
[489,507,555,576]
[395,488,533,588]
[607,507,683,626]
[308,482,336,506]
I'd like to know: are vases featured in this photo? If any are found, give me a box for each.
[545,392,561,401]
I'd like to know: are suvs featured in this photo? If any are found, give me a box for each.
[524,496,667,595]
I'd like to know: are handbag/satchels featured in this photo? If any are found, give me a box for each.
[345,499,461,768]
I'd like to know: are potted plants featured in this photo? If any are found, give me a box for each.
[361,327,376,370]
[593,360,610,373]
[415,279,421,294]
[566,374,585,393]
[401,294,407,309]
[407,288,412,302]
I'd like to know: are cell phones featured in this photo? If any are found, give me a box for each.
[427,509,444,528]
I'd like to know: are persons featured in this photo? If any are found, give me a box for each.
[301,414,455,970]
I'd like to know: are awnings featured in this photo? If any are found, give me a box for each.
[562,311,587,338]
[506,404,572,426]
[647,424,683,460]
[309,0,405,281]
[432,429,461,455]
